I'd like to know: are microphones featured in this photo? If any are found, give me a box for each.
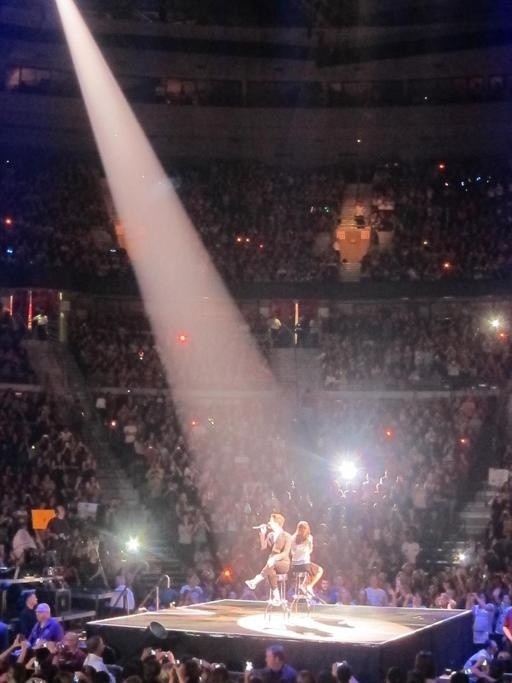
[252,524,270,530]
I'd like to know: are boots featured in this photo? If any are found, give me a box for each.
[245,574,264,589]
[272,587,281,607]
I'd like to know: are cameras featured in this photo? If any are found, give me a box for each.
[246,659,254,671]
[481,658,487,666]
[43,643,47,647]
[161,651,168,657]
[336,661,344,668]
[463,667,472,675]
[214,664,221,668]
[150,650,156,656]
[34,660,39,668]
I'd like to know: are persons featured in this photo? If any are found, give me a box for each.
[2,143,512,682]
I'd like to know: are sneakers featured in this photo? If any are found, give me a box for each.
[299,584,315,597]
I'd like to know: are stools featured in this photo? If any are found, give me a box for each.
[263,572,313,619]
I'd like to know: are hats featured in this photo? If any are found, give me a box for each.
[35,603,50,612]
